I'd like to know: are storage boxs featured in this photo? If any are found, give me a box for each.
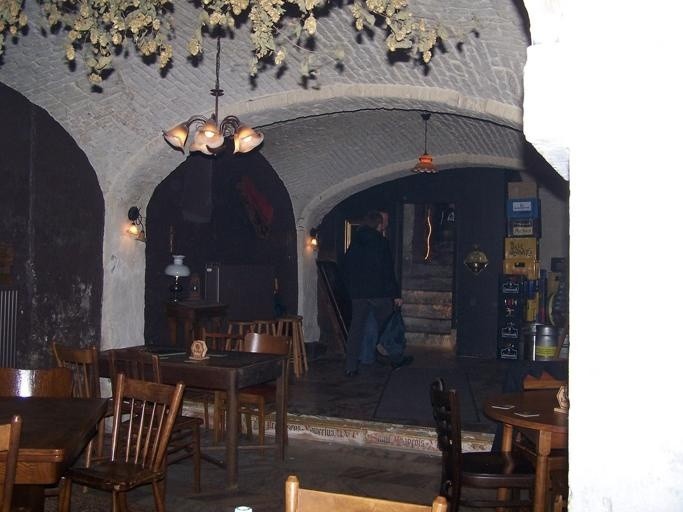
[502,181,543,282]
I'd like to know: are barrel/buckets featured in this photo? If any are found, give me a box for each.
[518,324,558,361]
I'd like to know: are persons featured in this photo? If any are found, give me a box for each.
[377,211,389,238]
[338,212,404,376]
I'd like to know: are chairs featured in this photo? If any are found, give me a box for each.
[284,476,447,512]
[210,333,289,455]
[62,374,187,511]
[50,336,138,468]
[1,415,26,512]
[1,367,72,510]
[110,351,202,502]
[427,378,551,512]
[513,380,568,512]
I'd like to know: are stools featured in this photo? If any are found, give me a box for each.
[285,315,309,374]
[224,320,255,352]
[252,319,278,336]
[277,318,302,381]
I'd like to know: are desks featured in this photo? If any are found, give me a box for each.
[482,390,569,512]
[166,303,228,348]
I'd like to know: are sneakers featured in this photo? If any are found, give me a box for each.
[345,368,362,377]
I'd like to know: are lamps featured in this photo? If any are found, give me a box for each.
[412,112,438,174]
[165,254,191,302]
[309,227,320,246]
[462,242,490,274]
[125,206,147,243]
[162,35,265,157]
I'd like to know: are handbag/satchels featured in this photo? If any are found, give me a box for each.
[374,305,407,369]
